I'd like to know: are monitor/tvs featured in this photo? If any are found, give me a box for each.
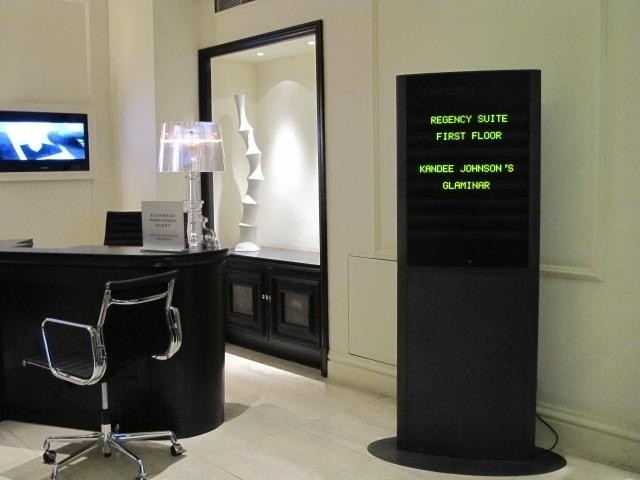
[0,111,89,172]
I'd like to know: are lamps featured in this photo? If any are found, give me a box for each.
[158,122,225,247]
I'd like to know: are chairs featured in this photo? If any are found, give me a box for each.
[103,211,187,247]
[20,270,187,479]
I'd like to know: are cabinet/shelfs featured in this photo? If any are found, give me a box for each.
[224,264,320,368]
